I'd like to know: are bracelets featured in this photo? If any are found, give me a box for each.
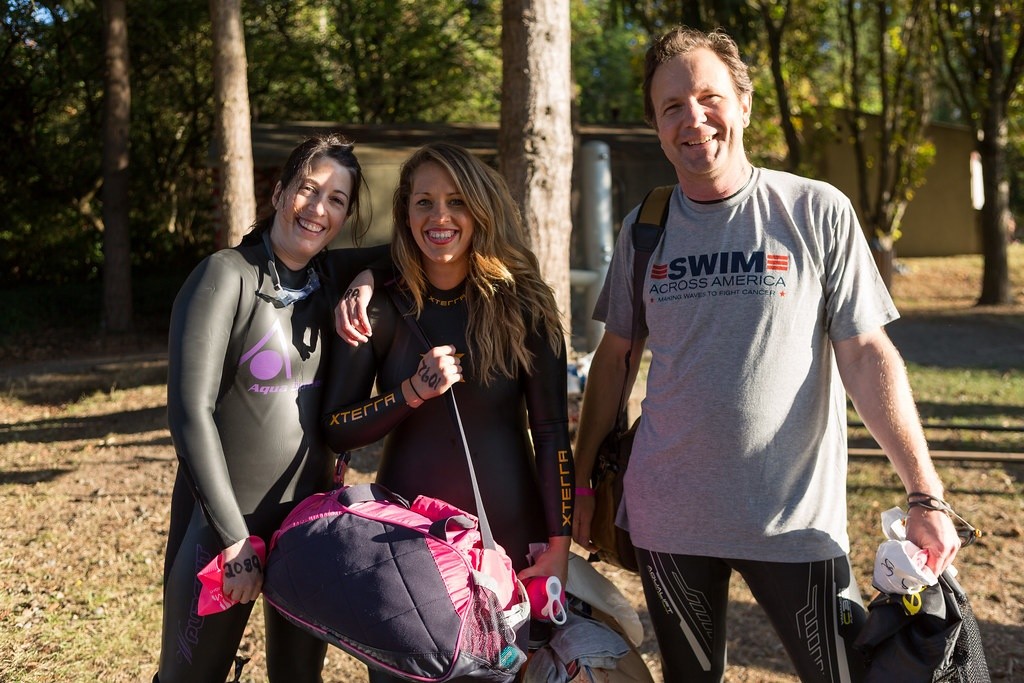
[576,487,595,496]
[409,377,427,402]
[907,492,951,511]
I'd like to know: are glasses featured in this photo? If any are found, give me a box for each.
[260,229,321,307]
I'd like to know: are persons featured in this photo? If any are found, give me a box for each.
[573,26,962,683]
[152,131,402,683]
[313,144,574,683]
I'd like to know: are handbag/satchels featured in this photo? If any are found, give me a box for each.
[853,566,991,683]
[589,415,642,573]
[257,485,531,683]
[516,540,654,683]
